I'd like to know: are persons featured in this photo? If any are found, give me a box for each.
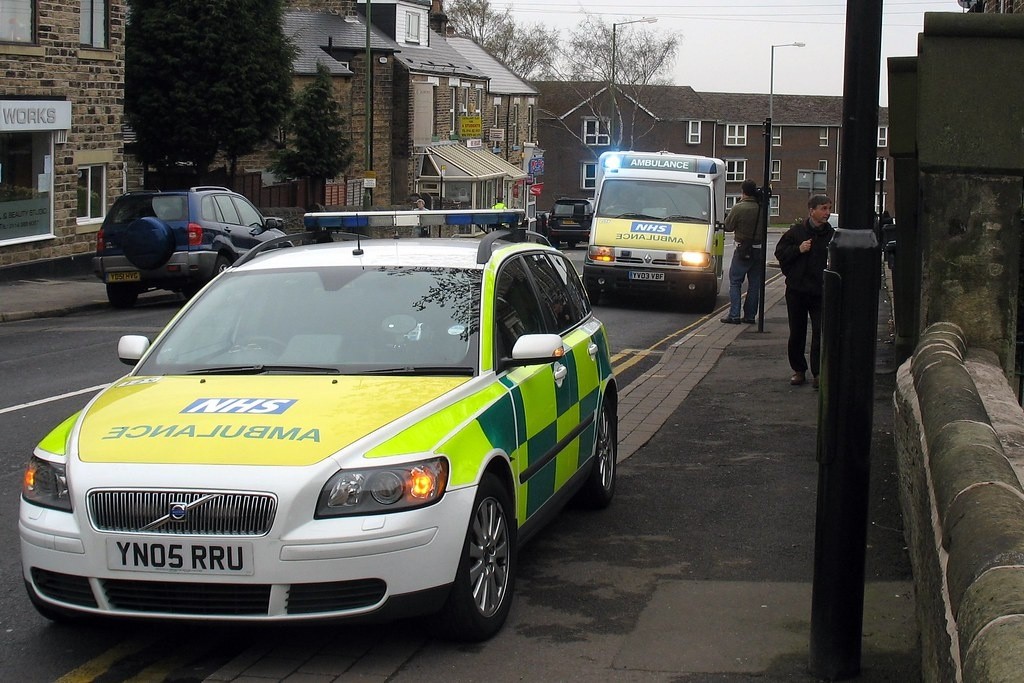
[720,179,764,324]
[774,195,836,389]
[491,197,507,231]
[552,298,571,327]
[411,199,430,238]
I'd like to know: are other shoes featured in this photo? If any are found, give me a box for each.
[813,375,820,388]
[721,317,740,324]
[790,372,804,384]
[741,318,755,324]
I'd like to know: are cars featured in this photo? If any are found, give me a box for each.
[536,210,550,245]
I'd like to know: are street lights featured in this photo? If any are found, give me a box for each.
[610,16,658,151]
[766,42,808,229]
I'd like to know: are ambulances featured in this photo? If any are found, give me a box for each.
[17,208,619,646]
[580,150,728,313]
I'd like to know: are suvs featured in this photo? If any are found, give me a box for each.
[89,184,294,312]
[547,198,593,249]
